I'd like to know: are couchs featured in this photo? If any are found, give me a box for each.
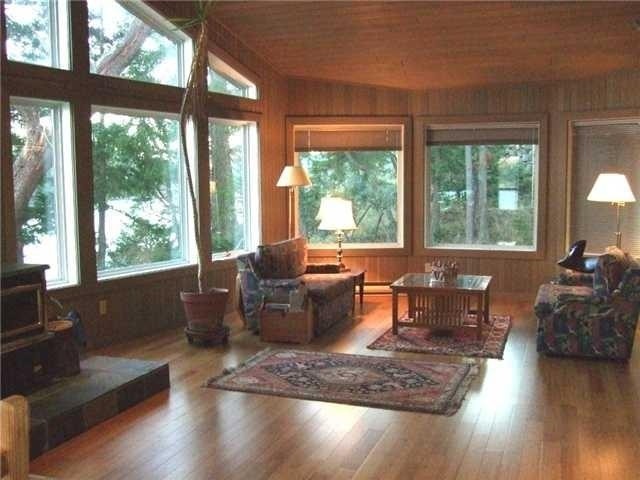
[534,246,639,362]
[237,235,355,336]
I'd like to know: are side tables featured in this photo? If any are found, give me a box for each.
[352,271,364,304]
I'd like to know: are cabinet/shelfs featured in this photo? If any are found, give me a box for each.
[257,296,313,345]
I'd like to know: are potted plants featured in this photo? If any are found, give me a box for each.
[162,0,231,333]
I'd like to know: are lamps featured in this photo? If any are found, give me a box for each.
[318,199,359,272]
[586,173,636,248]
[276,165,312,239]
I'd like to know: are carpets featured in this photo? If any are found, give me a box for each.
[199,346,481,418]
[366,306,513,359]
